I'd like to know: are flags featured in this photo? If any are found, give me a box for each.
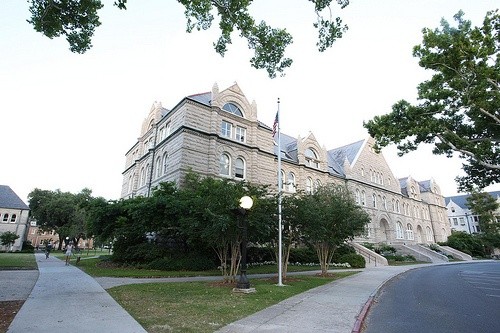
[272,111,278,138]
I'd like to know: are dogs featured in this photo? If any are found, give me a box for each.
[76,256,81,265]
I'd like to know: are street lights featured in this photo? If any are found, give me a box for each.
[235,196,254,289]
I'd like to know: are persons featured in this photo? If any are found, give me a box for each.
[64,242,73,267]
[44,242,51,258]
